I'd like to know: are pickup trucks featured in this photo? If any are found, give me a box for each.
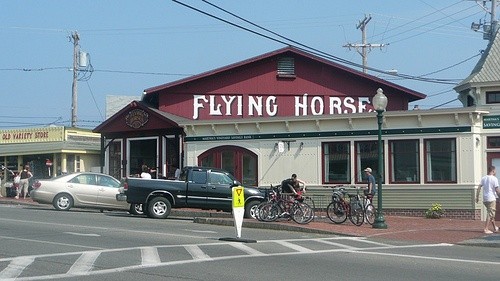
[116,165,267,219]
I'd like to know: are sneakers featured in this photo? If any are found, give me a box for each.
[493,227,498,232]
[484,229,493,234]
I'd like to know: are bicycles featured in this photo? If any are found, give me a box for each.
[254,184,378,226]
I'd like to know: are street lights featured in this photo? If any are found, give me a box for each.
[371,87,389,229]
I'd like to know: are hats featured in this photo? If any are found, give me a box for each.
[364,167,372,172]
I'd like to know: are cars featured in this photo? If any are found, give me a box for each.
[29,171,132,211]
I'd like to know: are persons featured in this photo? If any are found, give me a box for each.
[150,167,157,179]
[364,168,376,223]
[141,167,151,179]
[287,174,306,221]
[172,164,182,180]
[15,165,33,199]
[14,172,21,194]
[476,166,500,234]
